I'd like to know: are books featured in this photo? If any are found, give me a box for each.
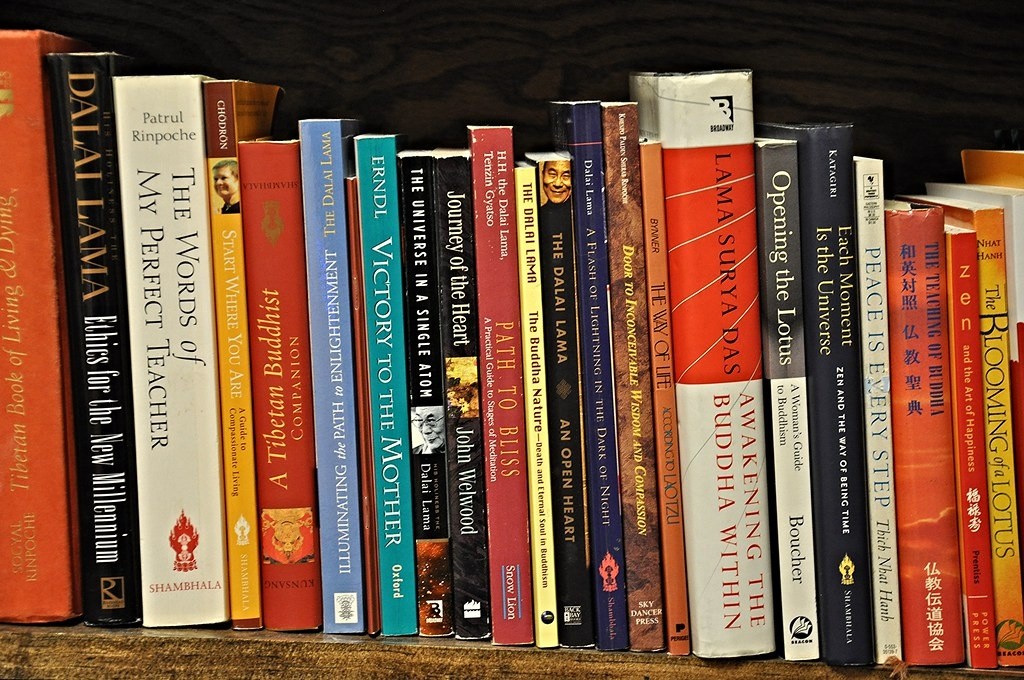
[0,27,1024,672]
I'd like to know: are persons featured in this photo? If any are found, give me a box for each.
[212,160,241,215]
[409,407,447,455]
[539,159,572,214]
